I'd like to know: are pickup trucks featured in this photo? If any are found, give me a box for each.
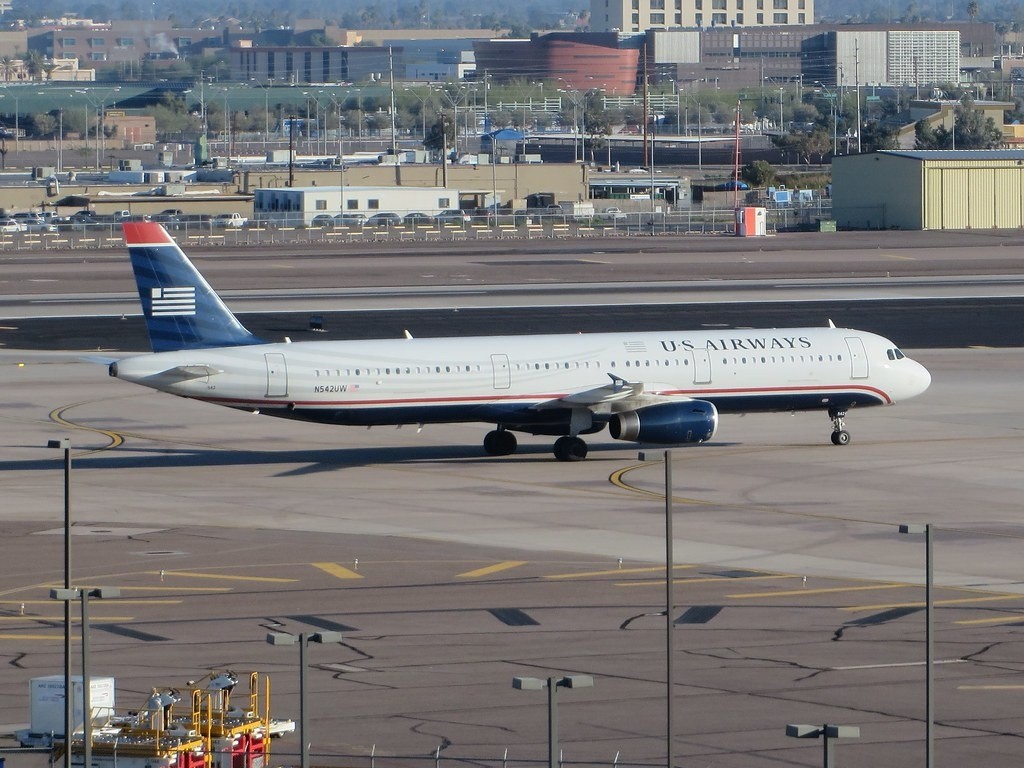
[212,213,248,227]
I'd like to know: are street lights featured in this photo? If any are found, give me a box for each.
[900,524,935,768]
[765,75,800,138]
[512,674,595,768]
[266,631,344,768]
[639,451,674,768]
[47,438,72,768]
[50,587,121,768]
[785,723,861,768]
[814,80,837,155]
[182,75,494,157]
[934,87,973,151]
[977,70,994,100]
[557,76,607,164]
[74,86,122,171]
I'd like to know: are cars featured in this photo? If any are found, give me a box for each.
[598,208,627,220]
[1,209,217,231]
[312,207,496,227]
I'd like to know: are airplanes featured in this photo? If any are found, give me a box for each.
[108,221,933,464]
